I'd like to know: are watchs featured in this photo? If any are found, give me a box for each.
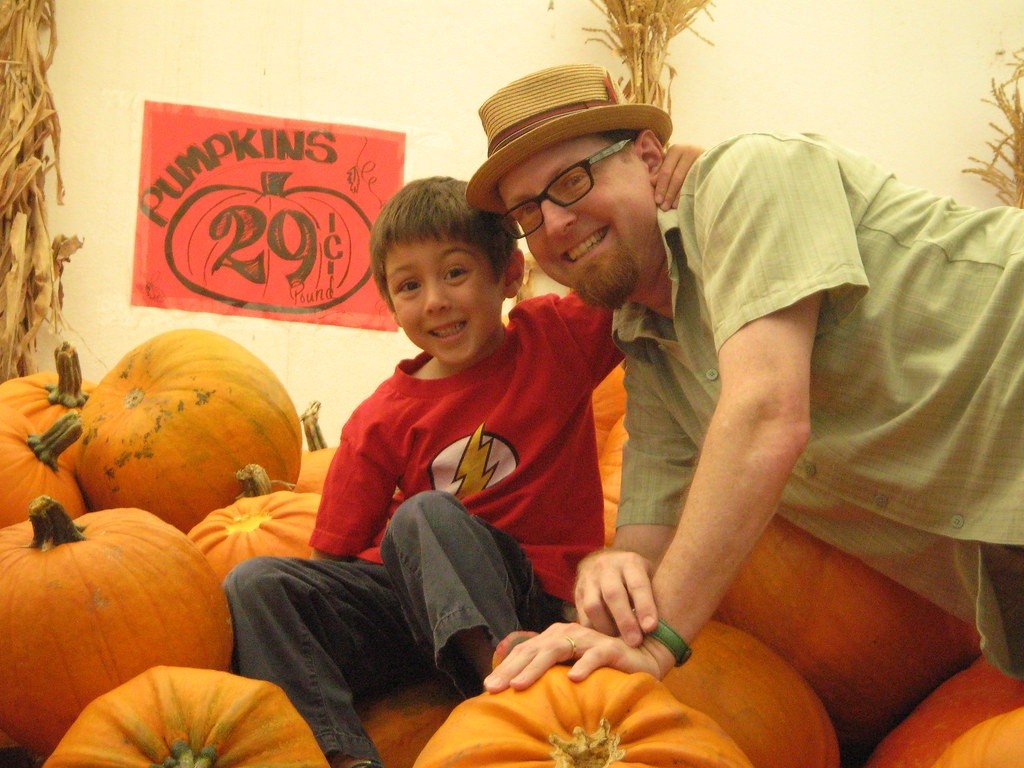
[631,607,692,666]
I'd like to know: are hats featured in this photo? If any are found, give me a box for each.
[463,62,674,218]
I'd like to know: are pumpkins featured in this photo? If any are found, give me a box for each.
[0,327,1023,768]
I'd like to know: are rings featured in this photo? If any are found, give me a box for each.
[566,637,576,661]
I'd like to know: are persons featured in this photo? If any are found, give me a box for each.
[474,66,1022,692]
[223,145,709,767]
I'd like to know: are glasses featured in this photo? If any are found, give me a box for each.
[499,136,634,239]
